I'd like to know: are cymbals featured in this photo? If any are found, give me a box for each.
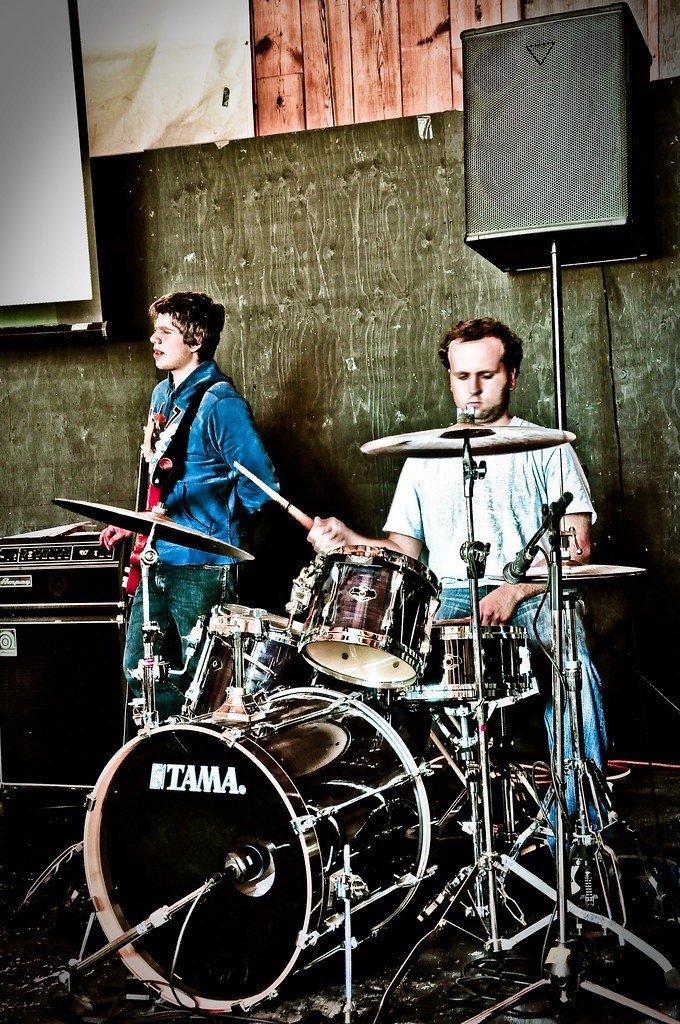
[50,496,256,561]
[484,559,647,586]
[359,423,576,458]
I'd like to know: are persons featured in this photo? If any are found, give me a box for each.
[98,291,281,726]
[304,315,611,905]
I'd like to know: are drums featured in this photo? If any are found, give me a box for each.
[83,687,431,1016]
[178,602,432,770]
[387,623,534,702]
[296,545,441,689]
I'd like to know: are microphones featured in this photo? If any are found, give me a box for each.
[503,546,540,585]
[224,844,270,882]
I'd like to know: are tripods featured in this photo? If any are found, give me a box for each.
[373,450,680,1024]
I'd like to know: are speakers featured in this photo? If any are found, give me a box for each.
[0,614,138,788]
[460,2,653,274]
